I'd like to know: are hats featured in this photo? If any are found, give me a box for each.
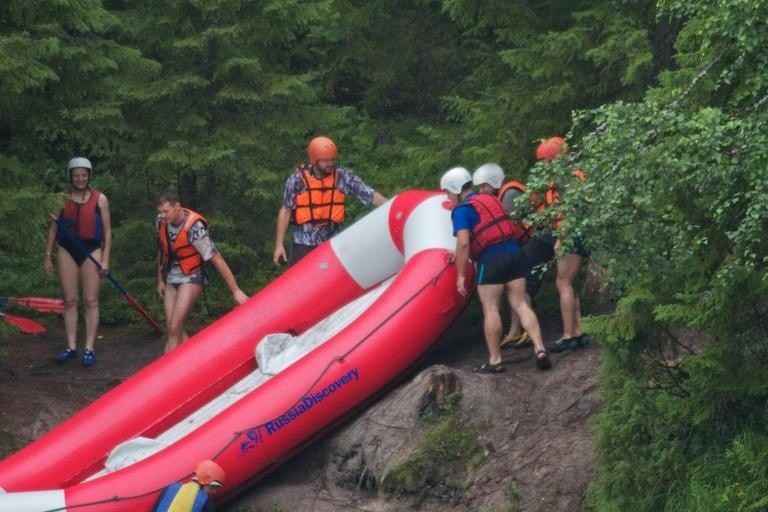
[192,475,223,487]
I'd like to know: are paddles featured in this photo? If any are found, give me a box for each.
[1,294,64,336]
[48,211,165,333]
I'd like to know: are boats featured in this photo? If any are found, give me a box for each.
[1,186,476,512]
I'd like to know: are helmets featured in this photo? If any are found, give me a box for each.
[195,461,225,485]
[472,163,505,189]
[309,137,337,165]
[537,137,566,162]
[440,166,473,195]
[67,157,92,182]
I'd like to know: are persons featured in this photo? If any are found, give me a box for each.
[43,157,110,366]
[440,168,552,372]
[274,137,387,268]
[473,163,554,348]
[151,460,225,511]
[156,192,249,356]
[536,136,588,353]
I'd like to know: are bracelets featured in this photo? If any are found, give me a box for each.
[44,251,51,256]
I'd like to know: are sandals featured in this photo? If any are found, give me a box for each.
[536,350,552,369]
[472,361,504,372]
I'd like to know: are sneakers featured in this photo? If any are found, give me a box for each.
[56,347,77,362]
[81,349,95,365]
[501,335,520,347]
[545,334,588,352]
[513,332,530,349]
